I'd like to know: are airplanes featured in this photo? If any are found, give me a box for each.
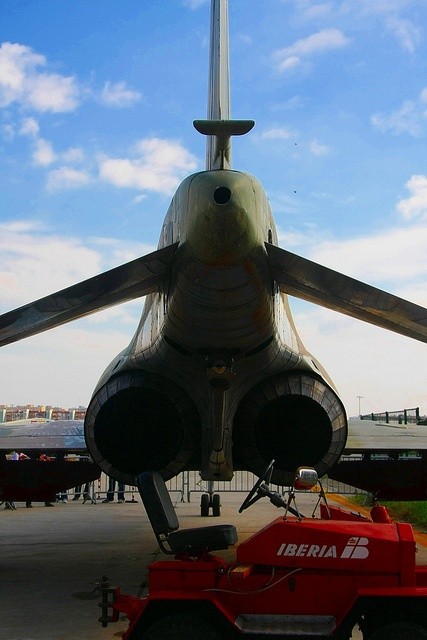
[0,0,427,555]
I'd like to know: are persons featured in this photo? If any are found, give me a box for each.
[72,482,91,501]
[55,490,68,504]
[101,477,125,504]
